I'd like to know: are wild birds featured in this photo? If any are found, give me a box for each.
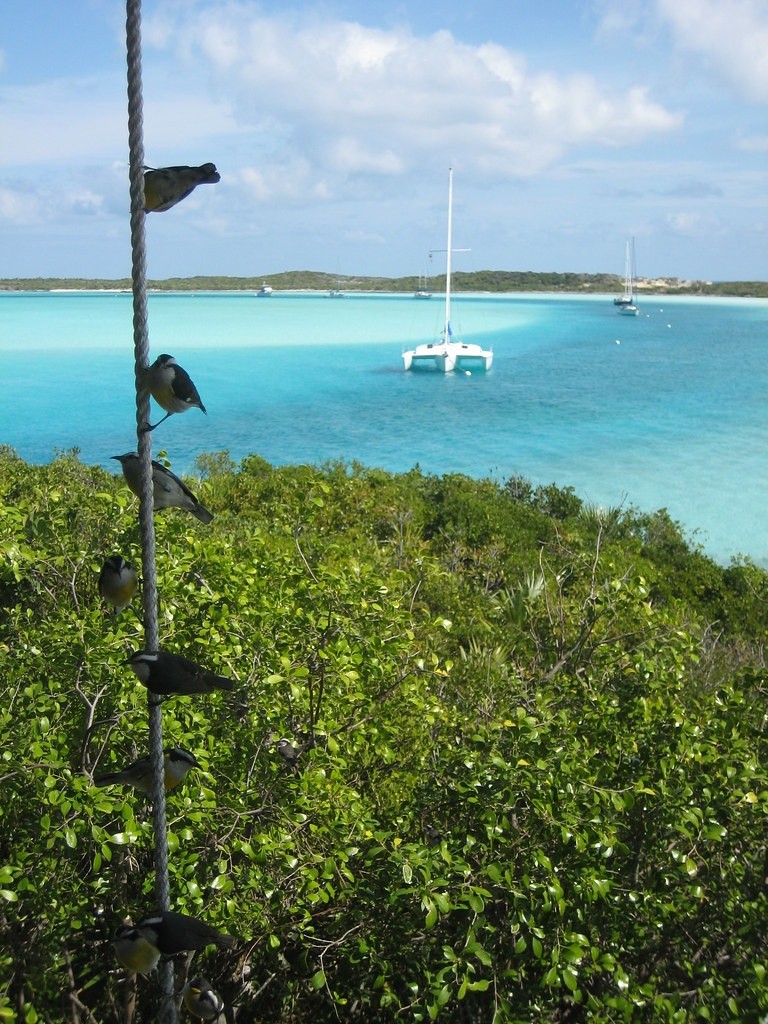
[143,354,207,432]
[93,747,205,802]
[129,162,221,214]
[277,738,300,780]
[119,650,234,707]
[98,556,138,614]
[185,979,222,1018]
[111,924,162,973]
[110,452,214,524]
[130,911,236,973]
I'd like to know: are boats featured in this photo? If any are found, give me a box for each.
[256,280,273,296]
[324,280,352,298]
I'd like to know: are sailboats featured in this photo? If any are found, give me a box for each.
[614,236,639,315]
[401,167,493,374]
[415,258,432,299]
